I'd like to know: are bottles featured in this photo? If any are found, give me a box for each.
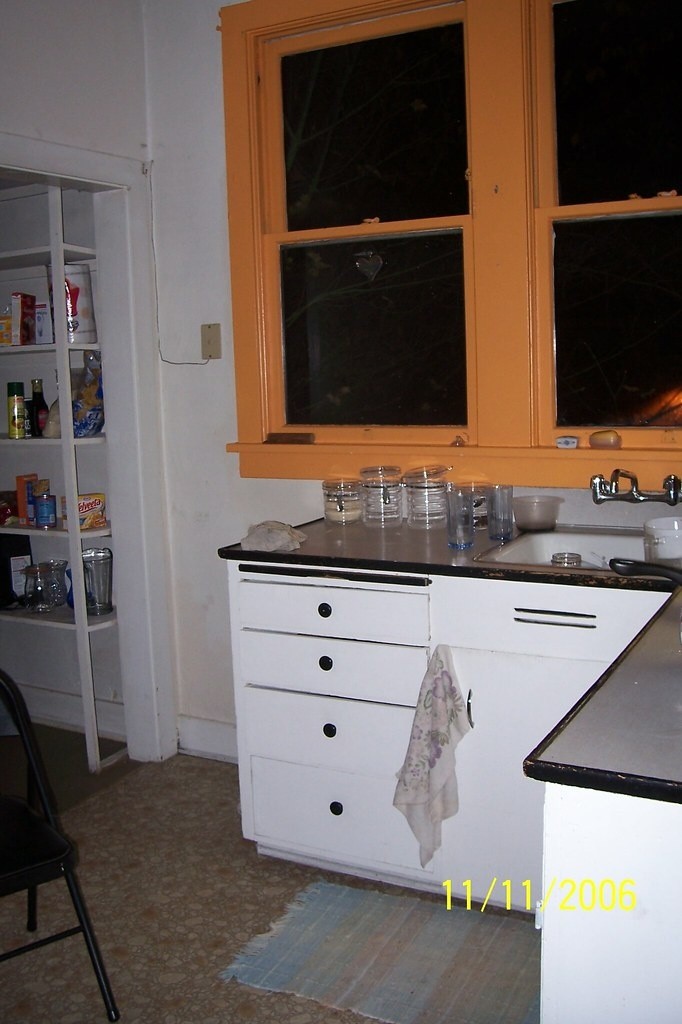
[551,552,581,567]
[399,465,453,530]
[450,480,494,535]
[322,478,361,527]
[29,379,49,436]
[360,466,403,529]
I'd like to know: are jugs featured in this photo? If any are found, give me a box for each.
[66,568,74,608]
[21,563,54,613]
[48,560,68,606]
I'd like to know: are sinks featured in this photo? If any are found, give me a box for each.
[473,529,646,575]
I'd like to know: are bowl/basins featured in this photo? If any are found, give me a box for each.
[512,495,564,532]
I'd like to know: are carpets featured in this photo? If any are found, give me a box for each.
[220,882,541,1024]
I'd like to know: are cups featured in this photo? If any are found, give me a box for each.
[445,492,475,550]
[487,484,513,541]
[644,516,682,559]
[82,548,113,616]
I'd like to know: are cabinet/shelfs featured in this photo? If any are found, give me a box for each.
[223,556,682,1024]
[0,173,128,772]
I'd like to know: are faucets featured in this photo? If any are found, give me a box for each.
[610,469,640,497]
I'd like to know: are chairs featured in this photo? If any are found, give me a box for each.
[0,669,120,1024]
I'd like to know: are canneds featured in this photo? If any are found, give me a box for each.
[34,494,57,528]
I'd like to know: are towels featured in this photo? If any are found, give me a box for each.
[393,645,470,870]
